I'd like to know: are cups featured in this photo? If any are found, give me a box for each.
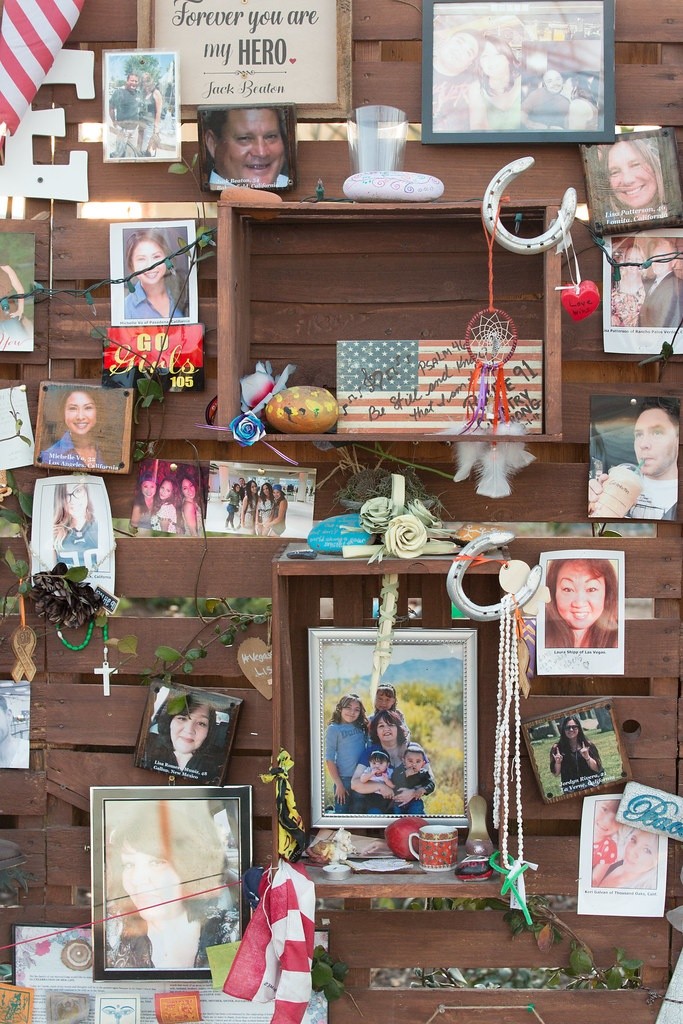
[408,825,458,871]
[345,104,409,176]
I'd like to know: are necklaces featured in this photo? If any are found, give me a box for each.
[493,594,523,871]
[55,615,119,697]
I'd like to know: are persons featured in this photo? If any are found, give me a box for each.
[358,838,388,856]
[589,398,678,520]
[40,386,108,470]
[50,483,99,571]
[591,800,659,890]
[132,472,289,536]
[545,558,617,647]
[104,800,240,968]
[0,265,34,339]
[0,696,29,768]
[548,715,605,796]
[326,683,435,814]
[124,229,189,319]
[598,138,665,225]
[613,239,683,327]
[143,692,219,781]
[109,72,163,158]
[435,27,601,130]
[203,106,290,190]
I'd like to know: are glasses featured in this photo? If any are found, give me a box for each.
[564,725,579,731]
[66,486,85,503]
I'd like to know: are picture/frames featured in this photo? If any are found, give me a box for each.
[520,699,633,805]
[197,103,297,194]
[420,1,616,144]
[89,785,252,978]
[34,380,133,475]
[134,681,245,788]
[136,1,354,123]
[583,129,682,235]
[307,625,480,830]
[102,48,181,164]
[0,216,51,365]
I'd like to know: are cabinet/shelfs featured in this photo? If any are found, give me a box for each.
[271,537,520,899]
[209,192,562,444]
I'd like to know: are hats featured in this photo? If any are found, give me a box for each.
[272,483,286,495]
[138,471,159,488]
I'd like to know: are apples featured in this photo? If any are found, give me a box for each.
[384,817,431,860]
